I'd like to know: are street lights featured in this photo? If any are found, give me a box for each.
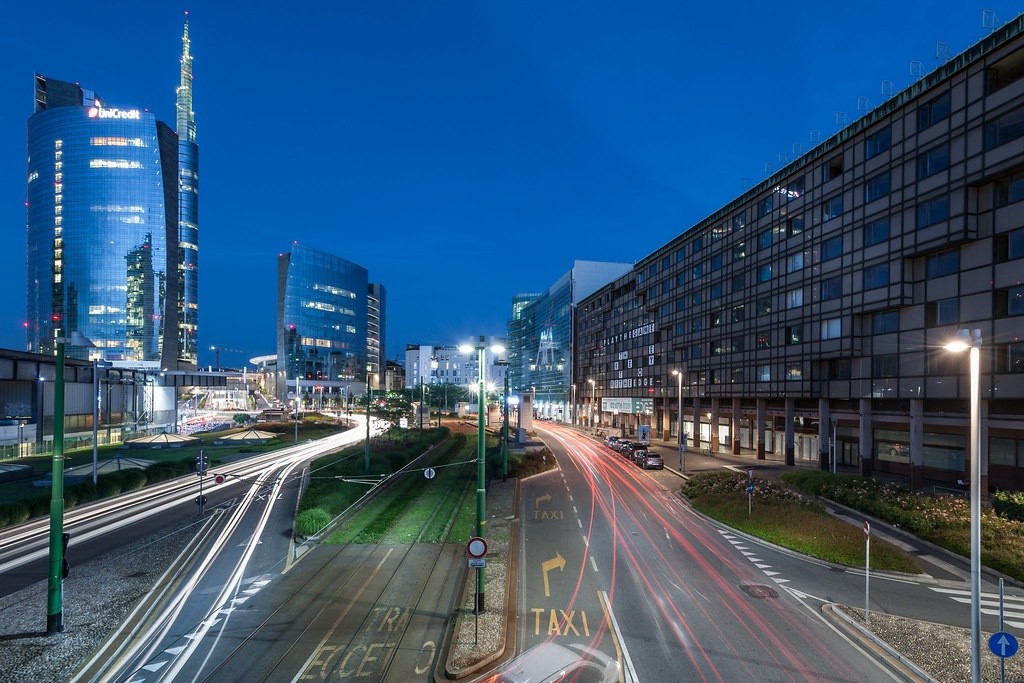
[531,386,535,419]
[93,359,113,484]
[943,329,982,682]
[672,370,682,471]
[317,385,324,412]
[459,336,506,615]
[346,384,351,426]
[47,330,96,634]
[589,379,595,439]
[706,413,712,456]
[295,375,304,445]
[570,384,576,427]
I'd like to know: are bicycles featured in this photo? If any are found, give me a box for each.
[703,446,715,457]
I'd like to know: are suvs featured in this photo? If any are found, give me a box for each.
[620,443,648,461]
[637,452,664,470]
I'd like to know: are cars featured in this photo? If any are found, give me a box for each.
[612,439,632,453]
[604,435,619,448]
[434,408,450,415]
[478,642,621,683]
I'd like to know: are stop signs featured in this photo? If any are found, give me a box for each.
[864,522,869,541]
